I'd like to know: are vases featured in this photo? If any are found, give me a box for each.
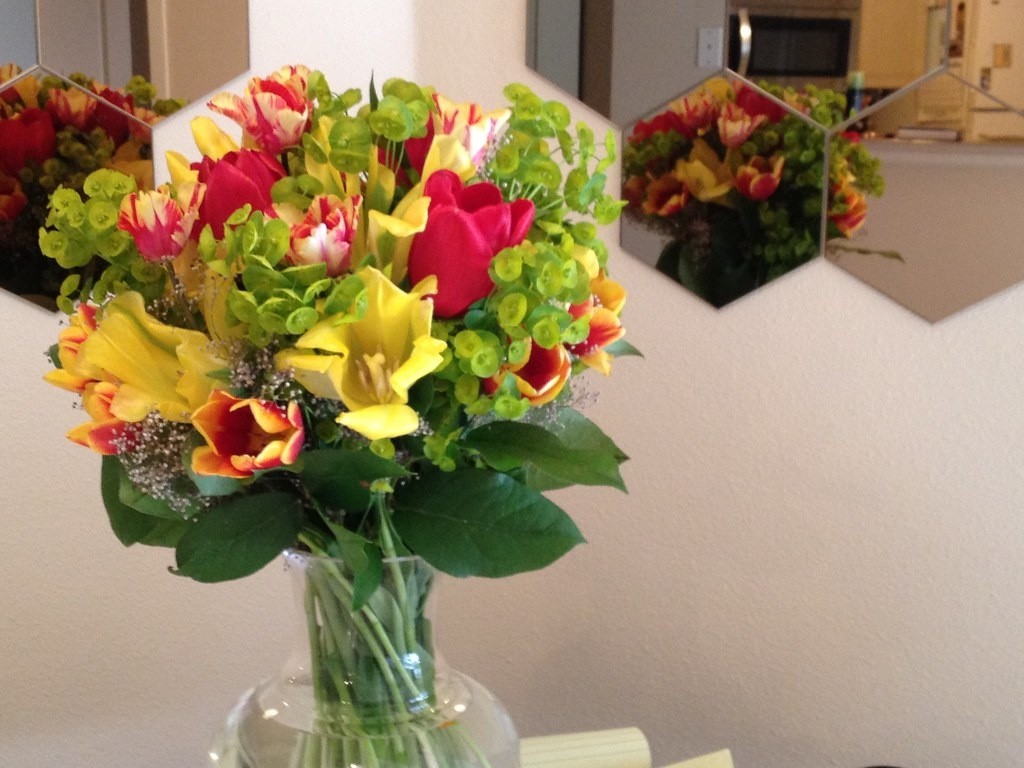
[208,548,521,768]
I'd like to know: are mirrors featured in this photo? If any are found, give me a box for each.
[0,0,253,314]
[522,0,1024,327]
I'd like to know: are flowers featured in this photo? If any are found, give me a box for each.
[31,63,649,768]
[619,80,906,310]
[0,61,184,311]
